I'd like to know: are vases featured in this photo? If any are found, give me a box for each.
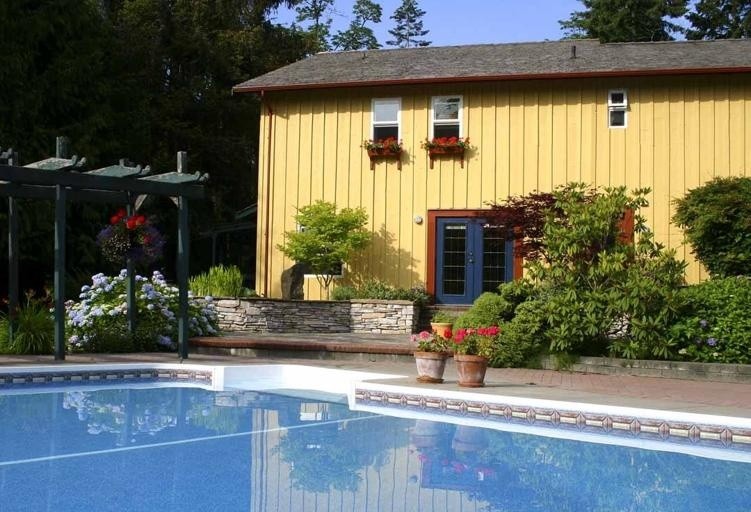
[411,349,489,388]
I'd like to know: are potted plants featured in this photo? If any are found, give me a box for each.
[429,311,458,338]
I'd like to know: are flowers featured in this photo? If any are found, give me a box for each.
[63,208,218,351]
[355,133,472,158]
[407,322,502,356]
